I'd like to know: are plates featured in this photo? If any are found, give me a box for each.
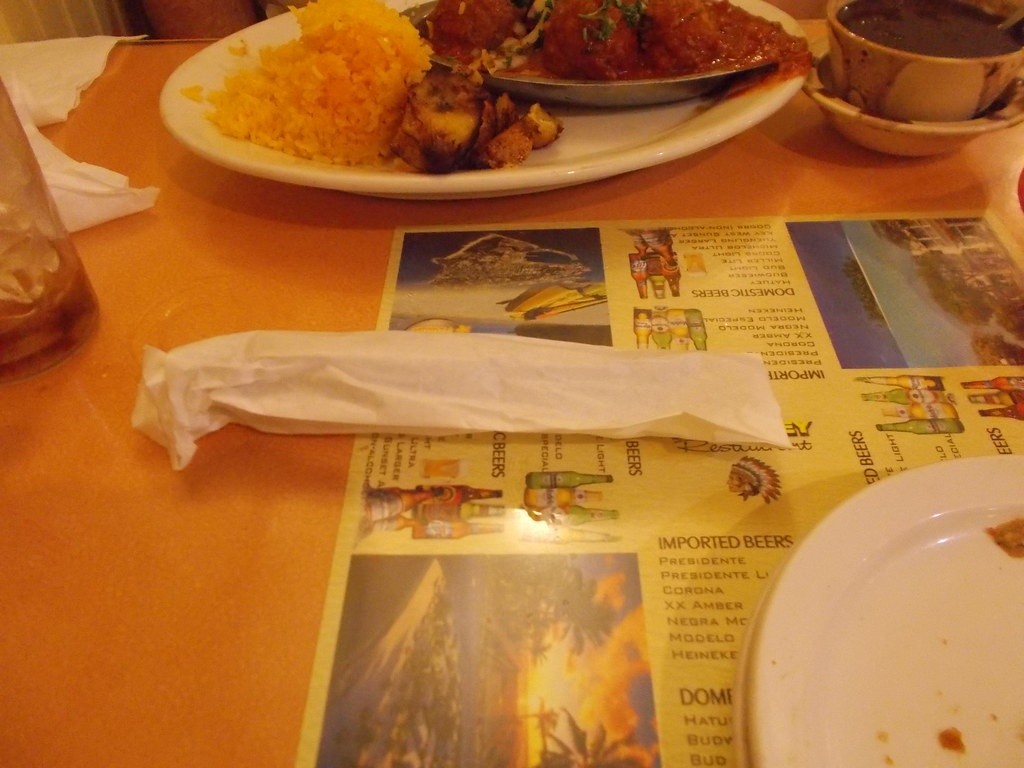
[395,0,783,107]
[157,0,807,202]
[745,453,1024,768]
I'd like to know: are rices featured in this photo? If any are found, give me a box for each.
[180,0,437,167]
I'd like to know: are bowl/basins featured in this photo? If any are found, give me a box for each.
[827,0,1024,115]
[803,36,1024,158]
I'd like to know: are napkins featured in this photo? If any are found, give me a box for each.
[0,33,163,234]
[133,332,795,470]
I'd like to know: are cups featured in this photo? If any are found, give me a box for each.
[0,79,101,383]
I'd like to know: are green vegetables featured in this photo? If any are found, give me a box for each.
[501,0,646,63]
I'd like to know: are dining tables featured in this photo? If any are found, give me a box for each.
[0,19,1024,768]
